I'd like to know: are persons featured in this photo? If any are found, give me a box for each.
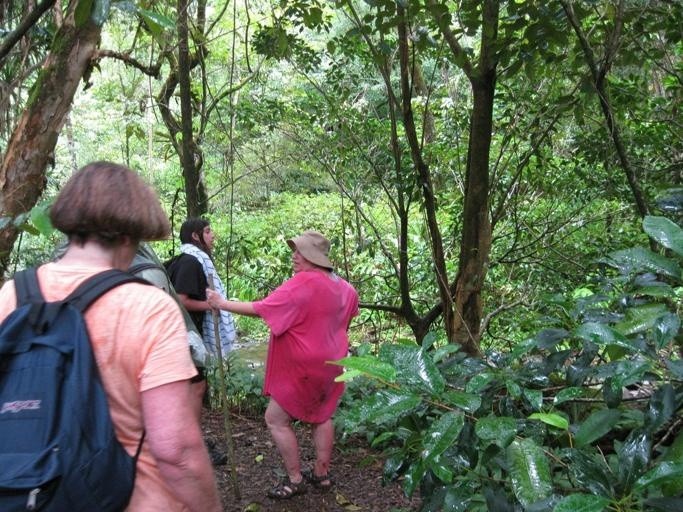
[203,230,361,502]
[0,158,229,512]
[40,225,209,372]
[162,216,233,466]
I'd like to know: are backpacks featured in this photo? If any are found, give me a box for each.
[0,266,156,512]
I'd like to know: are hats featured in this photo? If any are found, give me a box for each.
[286,231,333,272]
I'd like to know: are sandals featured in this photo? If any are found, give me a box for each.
[304,469,331,491]
[268,475,307,499]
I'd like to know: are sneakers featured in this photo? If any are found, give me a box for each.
[208,450,228,466]
